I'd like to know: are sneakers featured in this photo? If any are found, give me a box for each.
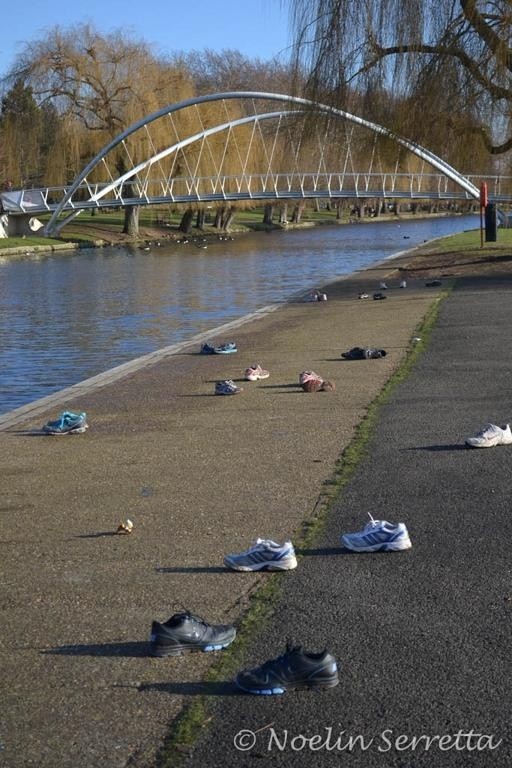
[150,603,235,658]
[342,347,386,359]
[245,364,270,381]
[215,379,243,395]
[358,290,386,300]
[303,291,327,302]
[299,370,334,392]
[466,422,512,447]
[342,513,412,552]
[400,281,407,288]
[425,281,442,287]
[214,342,237,354]
[236,638,338,694]
[43,411,89,436]
[223,537,297,572]
